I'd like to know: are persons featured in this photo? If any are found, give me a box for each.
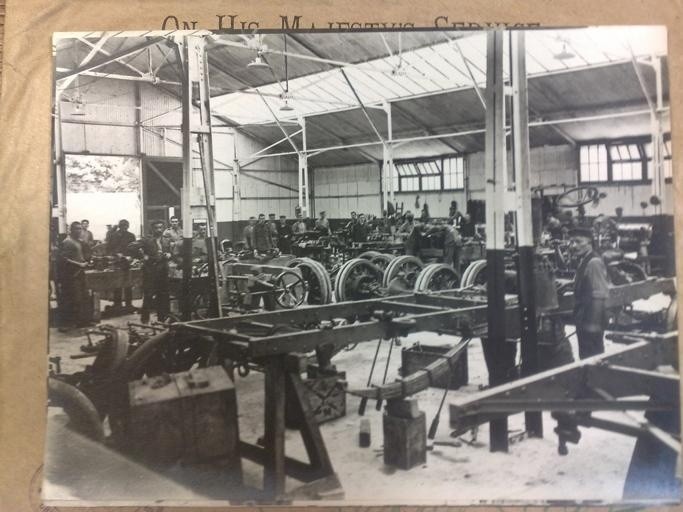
[243,201,476,273]
[192,223,209,259]
[126,218,172,324]
[541,208,621,251]
[564,225,608,360]
[161,215,184,263]
[51,221,100,335]
[78,219,94,244]
[108,218,137,312]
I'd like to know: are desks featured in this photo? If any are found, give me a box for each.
[85,269,143,319]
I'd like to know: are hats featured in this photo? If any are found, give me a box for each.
[567,228,593,238]
